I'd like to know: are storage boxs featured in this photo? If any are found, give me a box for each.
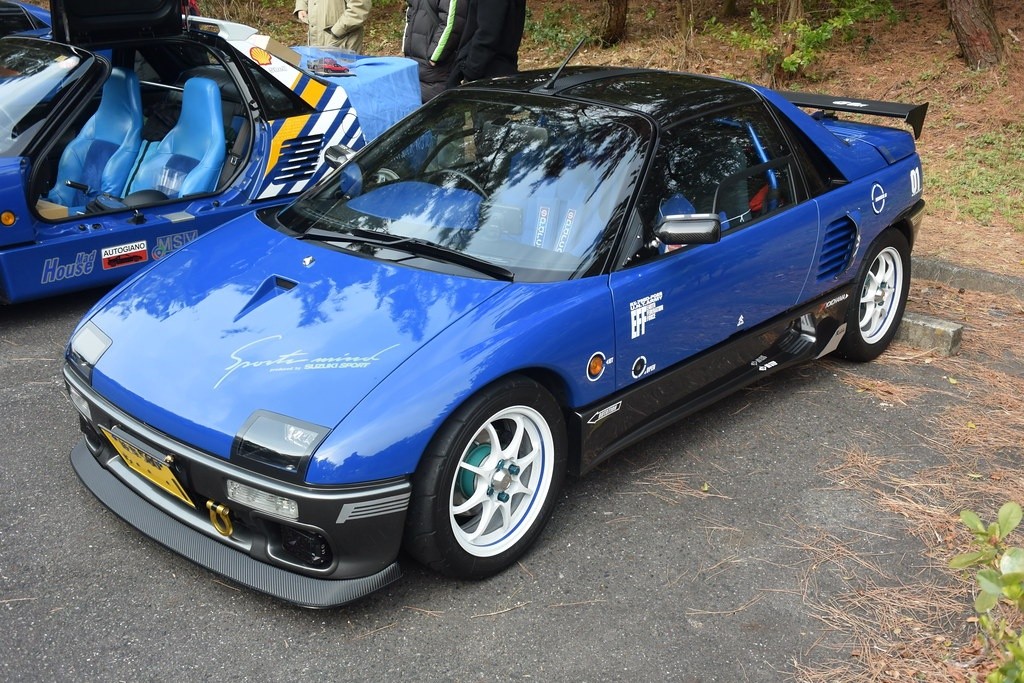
[248,35,302,66]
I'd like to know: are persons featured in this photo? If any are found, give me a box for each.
[292,0,372,55]
[401,0,526,175]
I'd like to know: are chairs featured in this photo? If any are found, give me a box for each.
[483,120,752,257]
[127,78,225,199]
[30,68,144,209]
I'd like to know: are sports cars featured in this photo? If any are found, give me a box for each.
[0,1,441,312]
[59,61,931,608]
